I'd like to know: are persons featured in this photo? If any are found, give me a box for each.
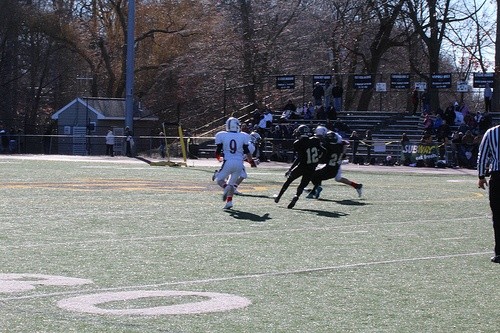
[211,117,257,209]
[241,83,492,210]
[0,129,52,155]
[477,125,500,263]
[122,126,132,158]
[105,129,114,157]
[159,132,165,159]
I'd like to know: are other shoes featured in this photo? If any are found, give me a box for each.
[316,187,323,199]
[273,194,279,203]
[223,202,233,209]
[305,193,313,198]
[212,170,218,181]
[490,255,500,263]
[232,185,241,195]
[287,197,298,209]
[356,183,363,198]
[222,184,233,201]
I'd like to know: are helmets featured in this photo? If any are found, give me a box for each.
[297,125,310,134]
[315,126,328,136]
[225,117,241,133]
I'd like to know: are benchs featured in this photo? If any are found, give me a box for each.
[199,111,500,156]
